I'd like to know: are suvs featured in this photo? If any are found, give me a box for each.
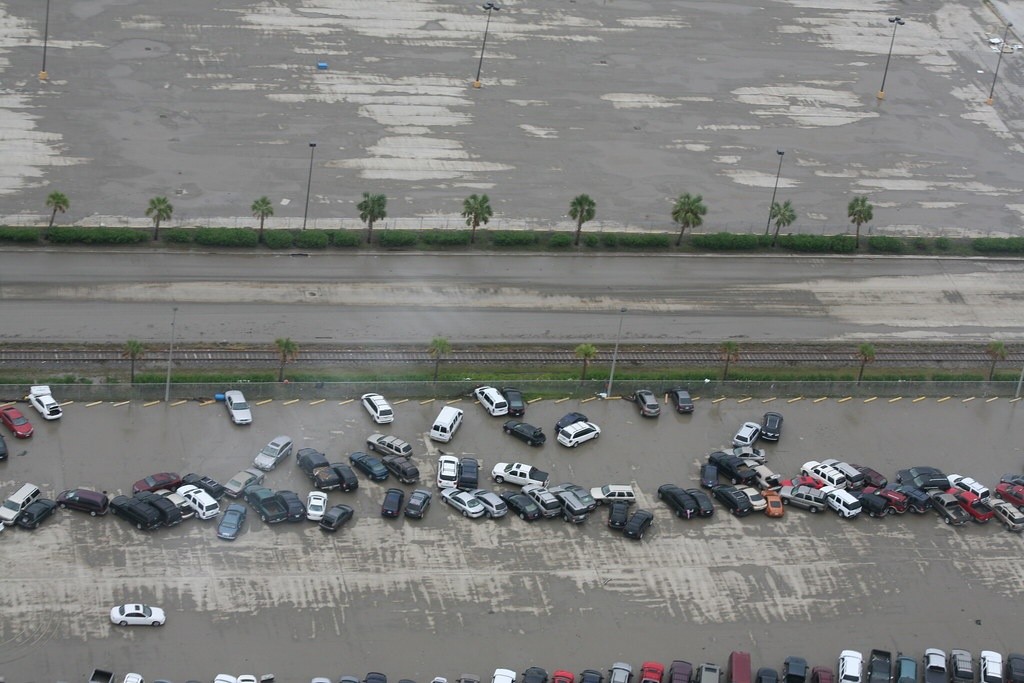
[557,421,602,448]
[429,406,464,444]
[473,385,509,417]
[361,392,395,424]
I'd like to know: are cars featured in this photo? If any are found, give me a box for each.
[500,386,526,417]
[554,412,589,434]
[761,411,784,442]
[502,419,546,447]
[670,389,695,414]
[634,389,660,417]
[1,402,1023,683]
[26,385,63,420]
[109,603,166,627]
[224,390,254,425]
[730,420,762,447]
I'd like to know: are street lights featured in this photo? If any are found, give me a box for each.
[880,14,906,94]
[989,22,1013,98]
[302,141,317,230]
[476,2,502,82]
[765,149,785,235]
